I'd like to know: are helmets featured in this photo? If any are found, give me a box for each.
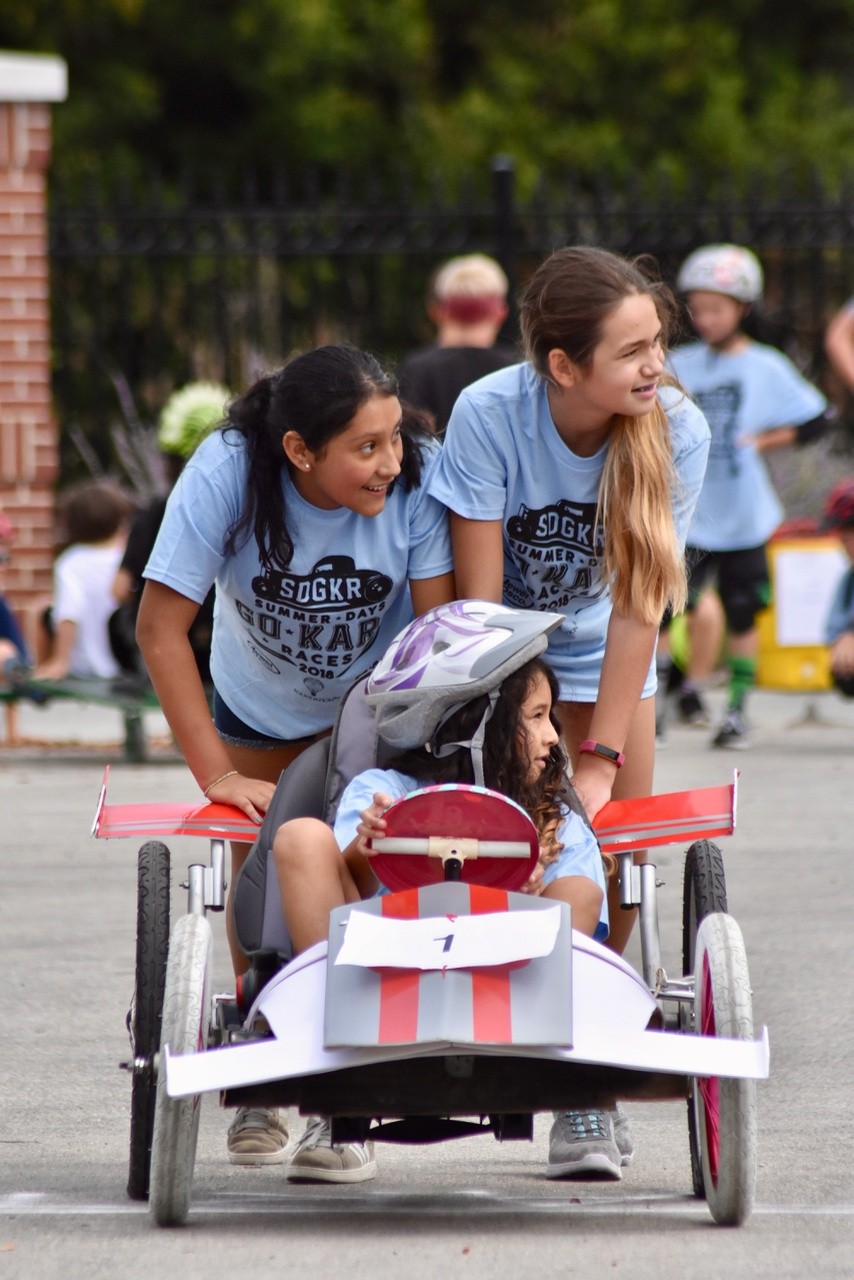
[677,243,762,305]
[364,598,567,751]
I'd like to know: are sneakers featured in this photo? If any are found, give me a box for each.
[547,1098,622,1180]
[288,1117,377,1183]
[611,1108,636,1162]
[227,1102,291,1165]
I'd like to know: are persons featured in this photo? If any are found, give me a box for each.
[391,256,523,434]
[0,383,246,697]
[438,247,708,1180]
[821,302,852,392]
[135,347,453,1182]
[653,243,838,747]
[272,600,607,962]
[816,480,852,701]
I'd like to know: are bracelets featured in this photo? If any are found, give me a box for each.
[201,767,239,796]
[579,740,627,770]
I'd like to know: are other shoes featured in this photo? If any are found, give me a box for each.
[710,711,751,744]
